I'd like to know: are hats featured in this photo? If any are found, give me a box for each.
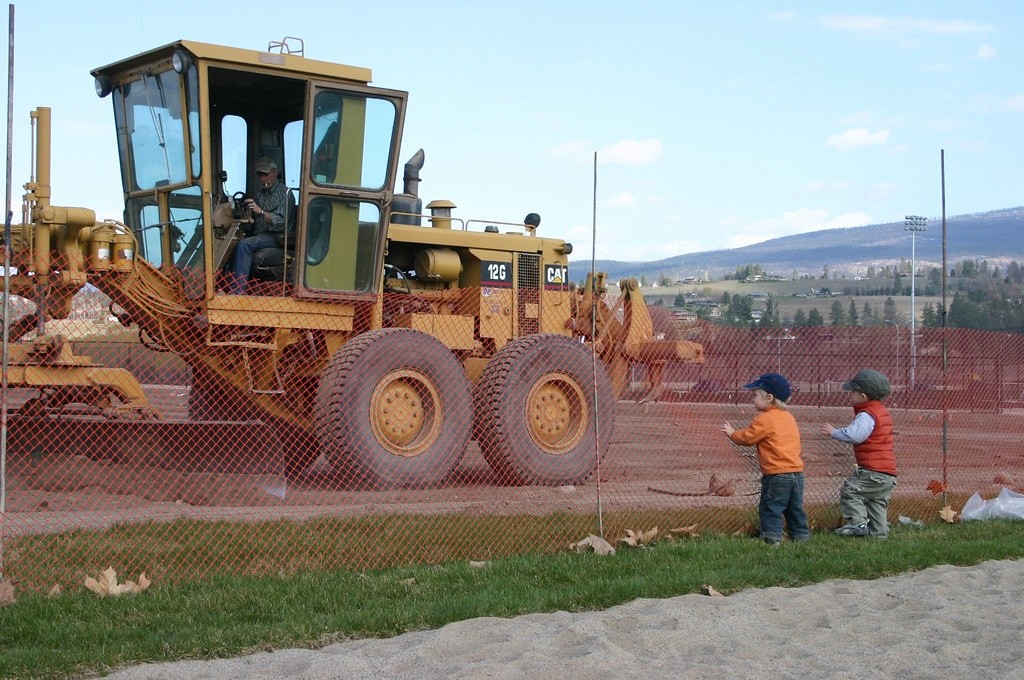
[842,370,890,399]
[255,156,277,173]
[744,373,790,402]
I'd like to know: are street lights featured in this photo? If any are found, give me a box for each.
[883,319,899,385]
[903,214,929,390]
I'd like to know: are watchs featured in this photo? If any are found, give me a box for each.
[258,209,266,218]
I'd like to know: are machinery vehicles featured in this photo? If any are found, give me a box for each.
[0,34,705,504]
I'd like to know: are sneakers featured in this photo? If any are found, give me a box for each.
[834,522,868,536]
[870,529,888,538]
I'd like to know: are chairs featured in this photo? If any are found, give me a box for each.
[248,205,302,282]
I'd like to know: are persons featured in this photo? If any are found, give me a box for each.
[819,369,898,542]
[224,156,295,296]
[720,372,811,545]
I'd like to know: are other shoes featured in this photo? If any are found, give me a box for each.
[762,537,781,546]
[788,533,811,543]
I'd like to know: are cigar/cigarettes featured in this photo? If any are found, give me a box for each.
[265,182,267,188]
